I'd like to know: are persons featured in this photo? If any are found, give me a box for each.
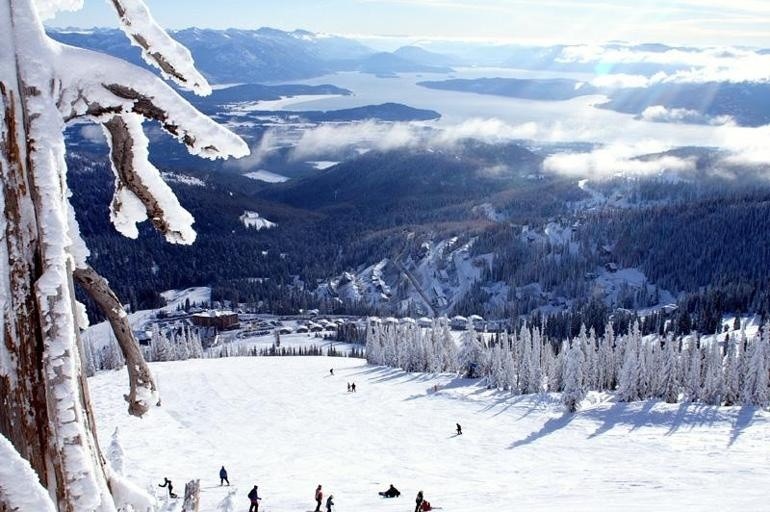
[352,382,356,391]
[348,382,350,391]
[158,477,180,497]
[247,485,262,511]
[326,494,335,511]
[415,491,423,512]
[219,465,230,484]
[420,499,433,511]
[384,483,399,498]
[330,368,334,374]
[315,484,323,512]
[456,422,462,434]
